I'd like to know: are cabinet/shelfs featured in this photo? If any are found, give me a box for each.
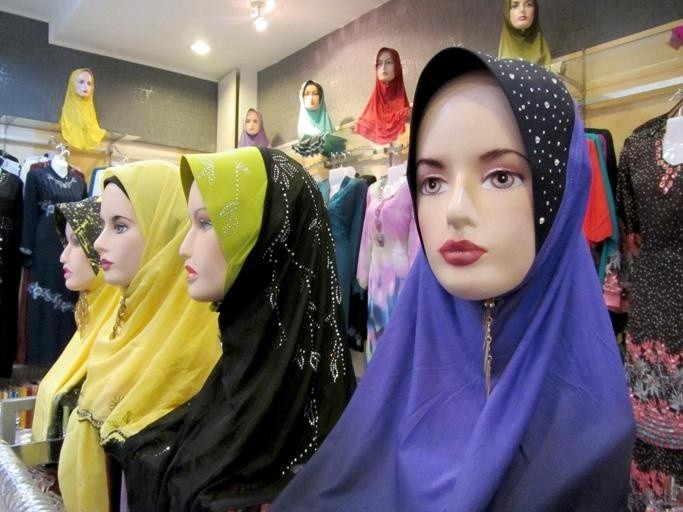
[0,393,64,448]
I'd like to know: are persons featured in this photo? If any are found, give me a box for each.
[298,80,333,136]
[124,147,357,512]
[33,193,122,439]
[499,0,550,69]
[355,48,410,145]
[60,69,106,149]
[58,160,223,512]
[270,48,636,511]
[240,108,269,146]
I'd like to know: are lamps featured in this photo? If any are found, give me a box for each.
[250,0,268,32]
